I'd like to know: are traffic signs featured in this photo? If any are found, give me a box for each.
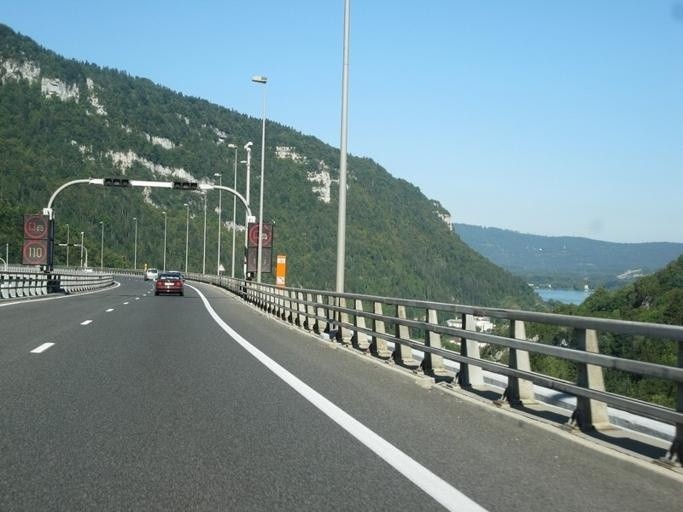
[22,239,47,266]
[248,248,271,273]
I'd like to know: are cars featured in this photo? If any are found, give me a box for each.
[152,273,183,296]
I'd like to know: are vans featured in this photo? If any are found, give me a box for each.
[143,269,158,281]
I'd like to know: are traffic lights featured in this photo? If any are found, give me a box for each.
[103,178,129,187]
[173,181,197,190]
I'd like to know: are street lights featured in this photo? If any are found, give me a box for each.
[5,243,9,267]
[56,201,190,275]
[200,191,207,276]
[214,171,222,278]
[250,73,268,284]
[226,144,238,279]
